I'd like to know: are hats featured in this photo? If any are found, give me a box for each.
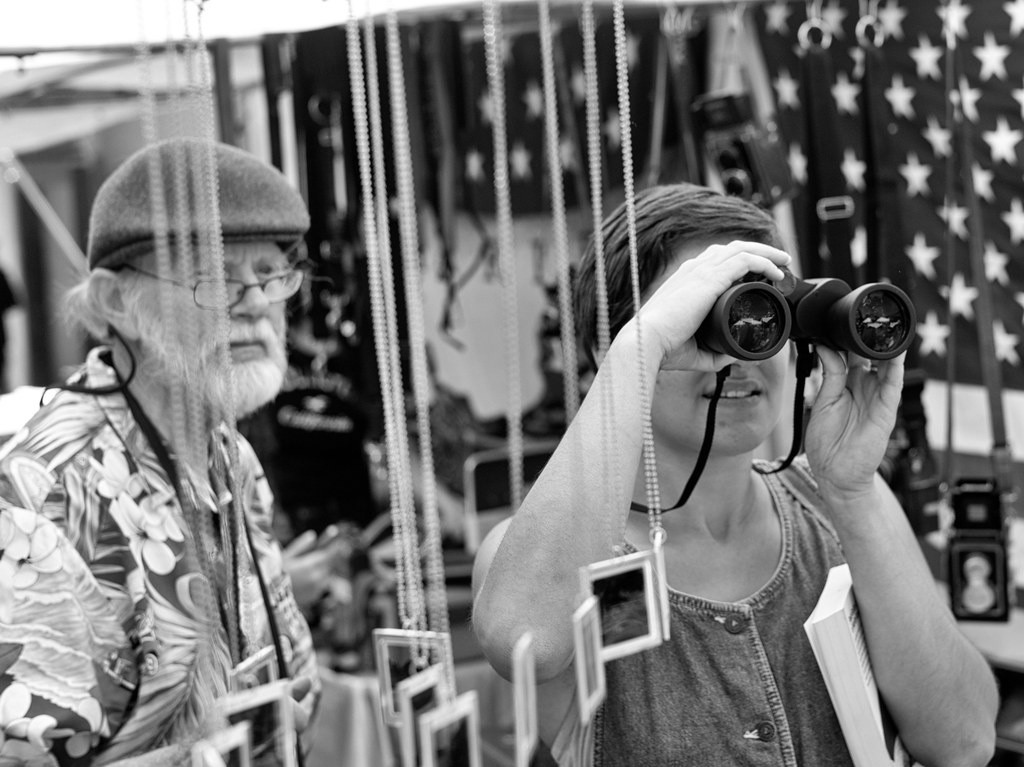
[87,138,310,270]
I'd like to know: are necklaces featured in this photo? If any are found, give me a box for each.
[110,365,291,681]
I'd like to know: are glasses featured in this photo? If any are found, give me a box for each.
[121,262,305,311]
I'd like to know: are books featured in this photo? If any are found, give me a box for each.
[803,564,910,767]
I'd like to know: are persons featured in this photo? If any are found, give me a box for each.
[0,136,322,767]
[474,183,999,767]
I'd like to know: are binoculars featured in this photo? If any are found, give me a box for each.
[700,266,919,361]
